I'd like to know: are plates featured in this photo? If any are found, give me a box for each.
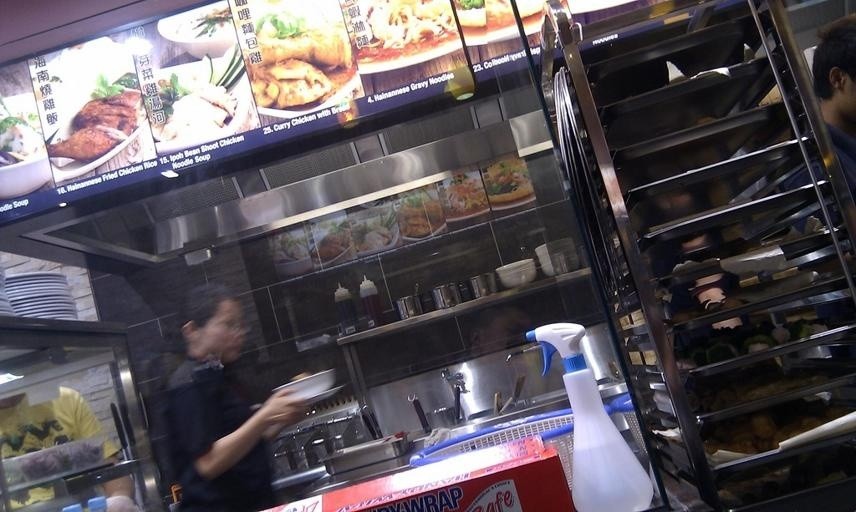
[303,385,346,405]
[463,12,547,47]
[400,223,447,241]
[257,69,361,120]
[0,92,53,201]
[138,56,251,154]
[350,206,400,258]
[50,114,148,183]
[311,226,352,269]
[446,208,491,223]
[357,38,463,75]
[0,266,77,319]
[491,197,537,211]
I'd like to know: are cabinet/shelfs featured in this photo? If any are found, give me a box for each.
[538,0,856,512]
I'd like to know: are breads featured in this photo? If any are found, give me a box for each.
[689,373,856,505]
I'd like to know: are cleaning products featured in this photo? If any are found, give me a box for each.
[524,323,655,512]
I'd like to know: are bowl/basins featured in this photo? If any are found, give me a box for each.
[535,237,580,277]
[274,255,313,275]
[495,259,537,289]
[158,0,236,60]
[271,368,336,400]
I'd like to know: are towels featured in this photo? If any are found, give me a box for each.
[422,425,454,447]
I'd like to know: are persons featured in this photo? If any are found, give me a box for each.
[770,7,855,373]
[156,282,310,512]
[0,382,141,512]
[639,161,782,424]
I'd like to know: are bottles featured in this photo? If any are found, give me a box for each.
[334,281,360,336]
[358,274,386,330]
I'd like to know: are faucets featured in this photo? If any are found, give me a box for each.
[440,367,469,420]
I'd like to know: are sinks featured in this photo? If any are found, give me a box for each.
[470,385,569,420]
